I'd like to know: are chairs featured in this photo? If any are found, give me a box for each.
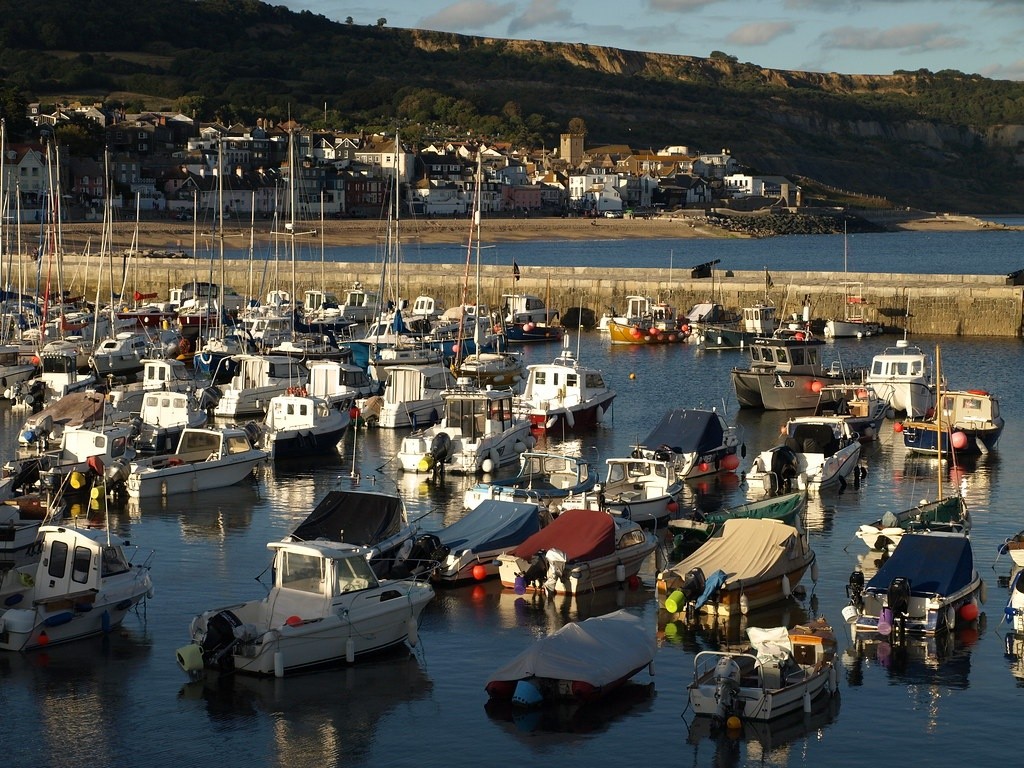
[475,415,485,434]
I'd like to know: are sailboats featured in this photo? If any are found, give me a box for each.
[0,120,839,519]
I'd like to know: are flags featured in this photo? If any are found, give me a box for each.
[514,262,520,280]
[766,272,774,289]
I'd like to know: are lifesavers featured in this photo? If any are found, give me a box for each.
[180,339,191,354]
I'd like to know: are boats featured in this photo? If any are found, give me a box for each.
[175,532,436,677]
[728,275,870,410]
[555,435,685,524]
[267,488,420,566]
[664,489,809,560]
[484,609,655,704]
[619,408,744,480]
[824,220,880,337]
[682,613,838,721]
[0,461,156,653]
[857,339,949,419]
[903,343,1005,458]
[989,529,1024,679]
[397,498,556,583]
[750,415,862,491]
[492,508,656,596]
[839,529,988,638]
[654,517,816,617]
[390,497,555,582]
[781,383,894,447]
